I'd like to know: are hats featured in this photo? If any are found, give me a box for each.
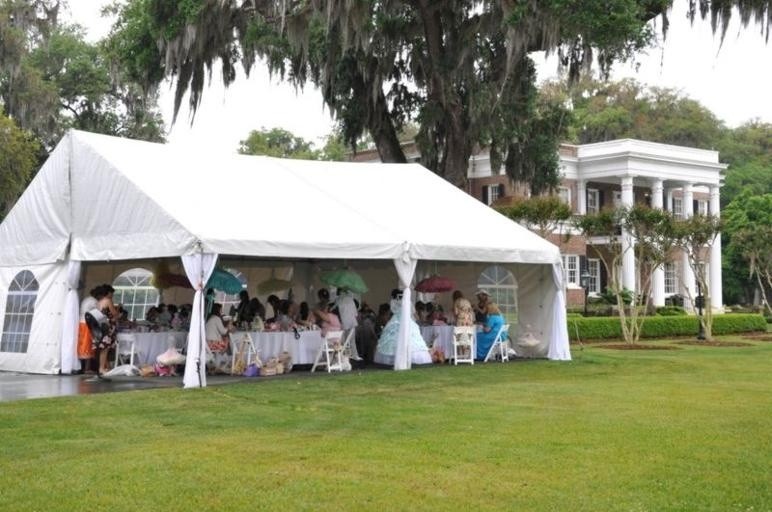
[475,289,492,297]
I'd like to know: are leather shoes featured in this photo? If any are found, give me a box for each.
[97,368,104,378]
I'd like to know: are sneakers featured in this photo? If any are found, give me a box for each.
[83,368,95,374]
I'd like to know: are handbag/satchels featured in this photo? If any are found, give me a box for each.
[234,353,293,376]
[141,362,182,377]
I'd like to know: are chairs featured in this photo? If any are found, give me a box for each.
[205,333,263,377]
[447,324,477,367]
[484,324,510,363]
[114,333,143,375]
[310,327,355,374]
